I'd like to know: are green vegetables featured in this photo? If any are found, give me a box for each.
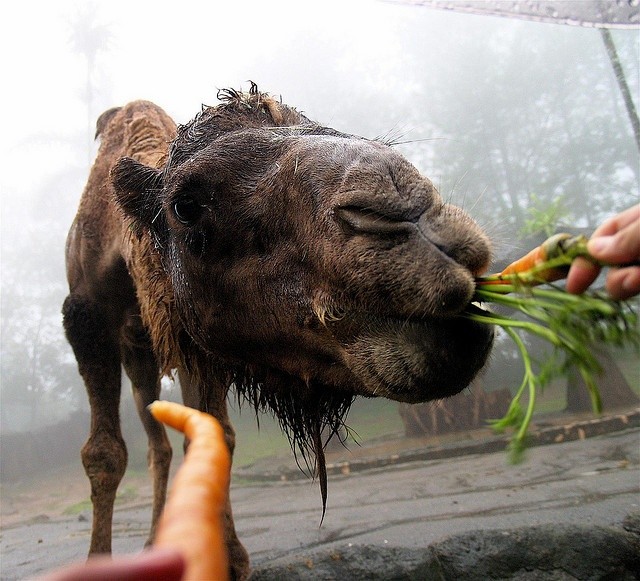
[470,285,639,465]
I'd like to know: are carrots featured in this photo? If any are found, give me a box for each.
[147,400,231,581]
[478,233,605,287]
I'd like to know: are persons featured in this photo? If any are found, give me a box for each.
[566,203,639,301]
